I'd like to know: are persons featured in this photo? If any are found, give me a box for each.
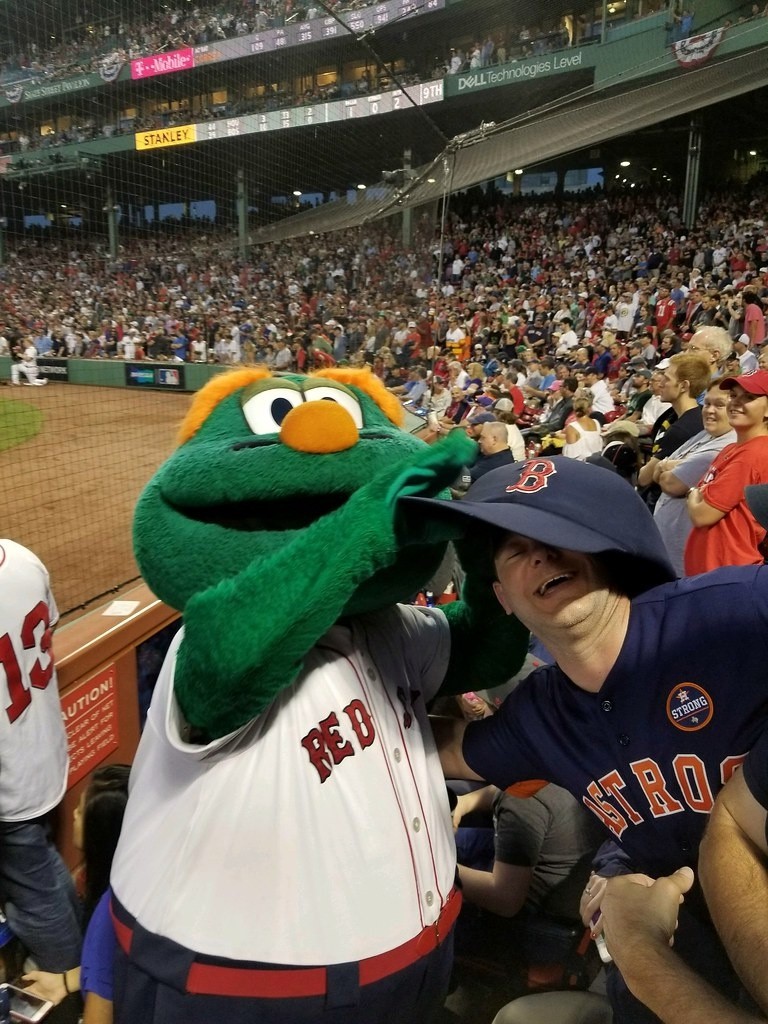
[393,454,768,1024]
[653,372,737,581]
[684,370,767,577]
[0,13,597,154]
[0,537,131,1024]
[0,0,381,83]
[671,2,767,39]
[0,180,768,510]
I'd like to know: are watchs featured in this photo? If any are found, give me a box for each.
[437,426,442,432]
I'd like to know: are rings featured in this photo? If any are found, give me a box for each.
[585,890,594,899]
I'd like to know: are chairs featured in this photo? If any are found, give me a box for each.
[459,909,604,997]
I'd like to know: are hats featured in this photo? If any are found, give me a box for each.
[623,292,633,300]
[732,333,750,346]
[474,343,482,350]
[578,292,588,299]
[720,365,768,398]
[744,484,768,530]
[130,321,138,326]
[548,380,561,391]
[467,413,496,424]
[476,396,493,406]
[636,369,652,379]
[398,454,679,593]
[408,321,416,327]
[485,398,514,412]
[655,358,670,370]
[627,356,644,365]
[325,320,336,325]
[552,332,561,337]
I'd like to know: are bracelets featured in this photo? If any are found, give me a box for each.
[659,456,669,472]
[63,970,72,995]
[686,487,696,495]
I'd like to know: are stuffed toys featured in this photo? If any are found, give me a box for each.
[107,366,532,1024]
